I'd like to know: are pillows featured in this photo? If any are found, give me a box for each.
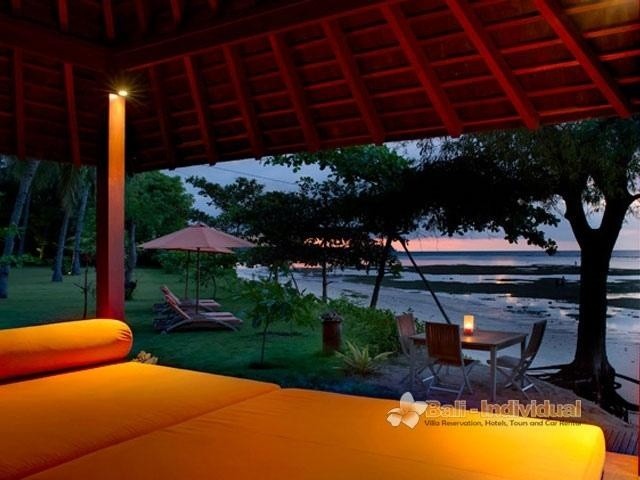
[0,316,137,379]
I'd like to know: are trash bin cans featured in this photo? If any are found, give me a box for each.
[320,316,343,353]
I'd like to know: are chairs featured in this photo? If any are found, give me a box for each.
[419,316,479,406]
[392,312,443,389]
[488,319,550,400]
[152,283,223,313]
[153,295,244,336]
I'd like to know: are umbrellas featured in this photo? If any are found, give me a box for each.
[134,225,236,302]
[143,219,260,315]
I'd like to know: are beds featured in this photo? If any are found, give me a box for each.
[1,358,609,480]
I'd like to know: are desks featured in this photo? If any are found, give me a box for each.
[404,327,533,409]
